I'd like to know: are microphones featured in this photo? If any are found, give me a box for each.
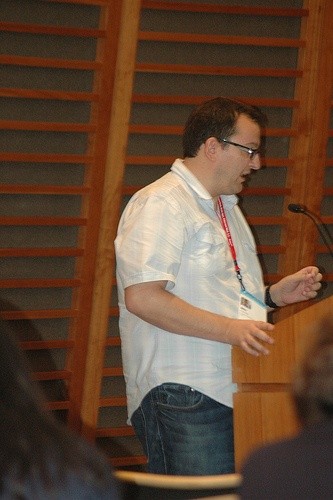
[288,203,332,258]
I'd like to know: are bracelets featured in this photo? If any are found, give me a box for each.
[265,285,280,308]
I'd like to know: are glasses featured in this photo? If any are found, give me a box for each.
[213,136,261,159]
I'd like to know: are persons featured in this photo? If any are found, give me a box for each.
[113,98,321,491]
[241,335,333,500]
[0,336,122,500]
